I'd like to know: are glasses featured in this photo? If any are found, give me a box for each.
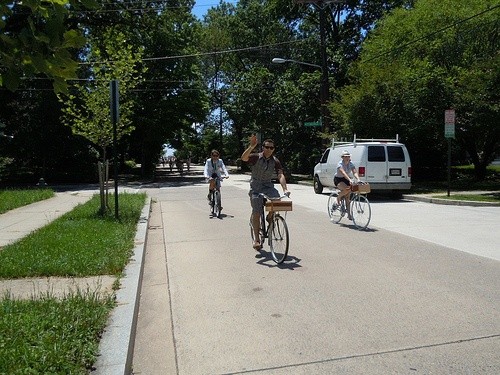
[262,144,275,149]
[212,154,219,157]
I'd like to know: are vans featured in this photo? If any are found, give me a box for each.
[312,133,412,200]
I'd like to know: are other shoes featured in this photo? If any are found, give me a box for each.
[252,239,263,249]
[208,194,210,200]
[348,215,352,220]
[266,215,276,228]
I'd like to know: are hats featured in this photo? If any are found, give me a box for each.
[340,149,350,158]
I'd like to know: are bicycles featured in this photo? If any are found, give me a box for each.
[249,191,291,265]
[327,180,372,230]
[169,164,190,175]
[205,174,229,218]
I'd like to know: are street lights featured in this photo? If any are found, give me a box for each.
[272,57,324,154]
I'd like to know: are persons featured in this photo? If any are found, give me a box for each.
[162,156,191,173]
[204,149,229,210]
[333,150,360,220]
[240,134,289,248]
